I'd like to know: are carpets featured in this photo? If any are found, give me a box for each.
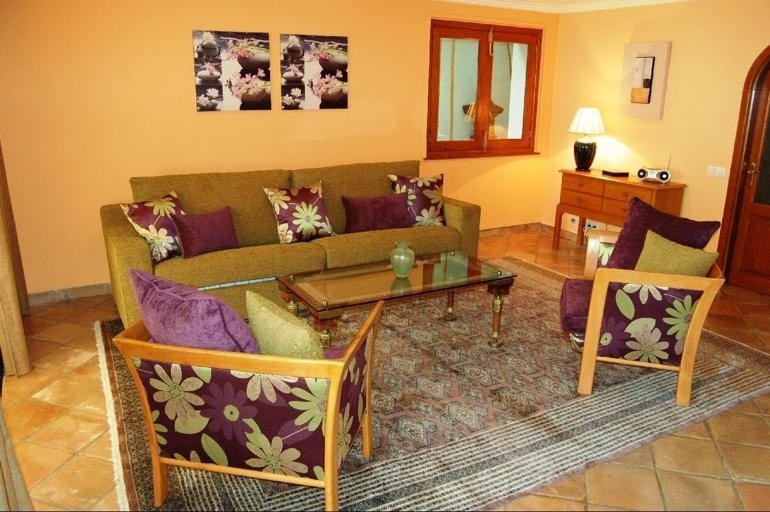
[91,258,770,512]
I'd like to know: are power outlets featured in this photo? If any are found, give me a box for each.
[587,222,599,229]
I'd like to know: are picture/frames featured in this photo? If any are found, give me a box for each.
[617,40,672,119]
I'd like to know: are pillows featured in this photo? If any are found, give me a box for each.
[632,231,720,279]
[130,268,264,354]
[601,198,721,267]
[263,181,335,245]
[119,191,189,263]
[389,172,447,228]
[169,208,241,259]
[341,191,413,233]
[245,291,326,360]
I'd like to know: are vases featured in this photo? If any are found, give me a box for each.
[389,277,413,295]
[390,239,415,279]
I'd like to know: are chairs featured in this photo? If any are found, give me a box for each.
[112,297,383,511]
[557,234,728,406]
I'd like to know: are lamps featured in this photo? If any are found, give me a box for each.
[569,106,602,171]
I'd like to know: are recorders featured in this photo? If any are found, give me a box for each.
[636,166,672,184]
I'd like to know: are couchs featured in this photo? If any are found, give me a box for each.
[99,158,482,331]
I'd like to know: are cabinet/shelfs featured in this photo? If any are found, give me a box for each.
[553,166,686,252]
